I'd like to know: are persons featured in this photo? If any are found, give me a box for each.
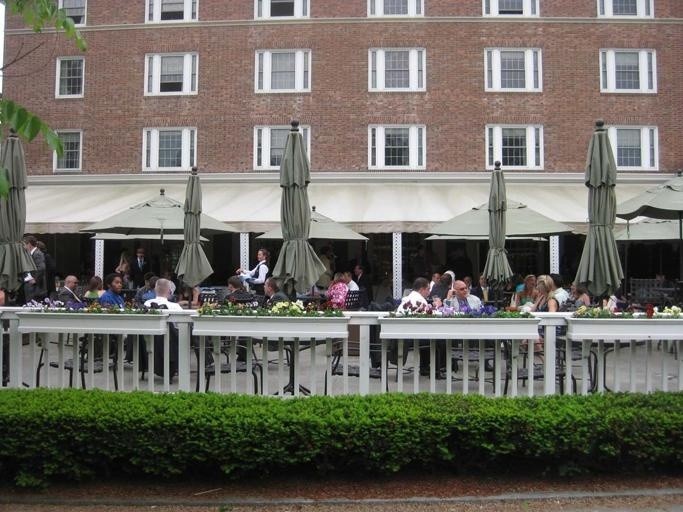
[391,270,628,379]
[20,237,373,366]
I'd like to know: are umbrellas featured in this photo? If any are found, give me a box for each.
[483,160,514,307]
[271,119,327,297]
[424,198,572,242]
[616,169,683,282]
[0,128,37,306]
[254,205,370,297]
[79,166,241,309]
[574,118,625,310]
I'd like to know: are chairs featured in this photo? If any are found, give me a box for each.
[32,273,683,397]
